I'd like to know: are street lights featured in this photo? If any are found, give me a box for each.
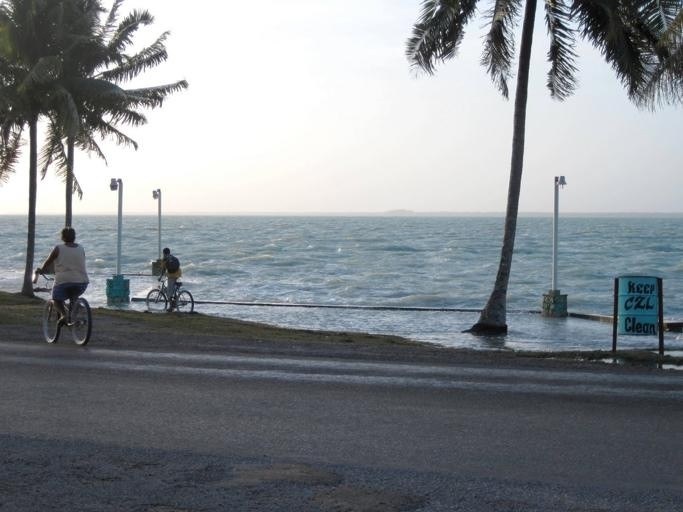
[551,173,567,289]
[151,187,161,258]
[109,176,122,274]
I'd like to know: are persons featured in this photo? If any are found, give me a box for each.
[152,246,181,308]
[35,227,90,327]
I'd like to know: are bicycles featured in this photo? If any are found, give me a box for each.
[30,267,91,345]
[146,279,194,315]
